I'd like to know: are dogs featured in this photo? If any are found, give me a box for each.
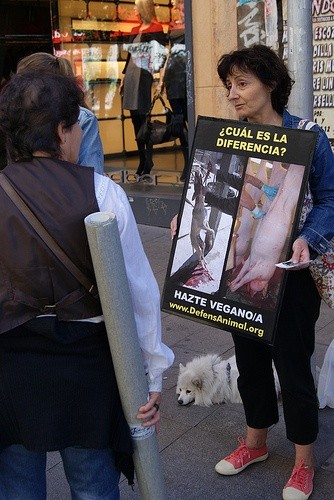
[176,351,243,408]
[190,170,215,271]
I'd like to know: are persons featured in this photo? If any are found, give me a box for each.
[1,70,176,500]
[152,0,185,182]
[201,155,247,219]
[15,53,106,178]
[169,44,334,500]
[239,174,279,219]
[118,0,166,179]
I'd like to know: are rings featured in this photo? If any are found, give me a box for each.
[154,403,160,411]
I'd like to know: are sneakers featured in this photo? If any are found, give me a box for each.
[215,435,268,476]
[282,458,314,500]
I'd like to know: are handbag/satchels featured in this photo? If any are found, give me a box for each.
[135,93,184,146]
[295,119,334,312]
